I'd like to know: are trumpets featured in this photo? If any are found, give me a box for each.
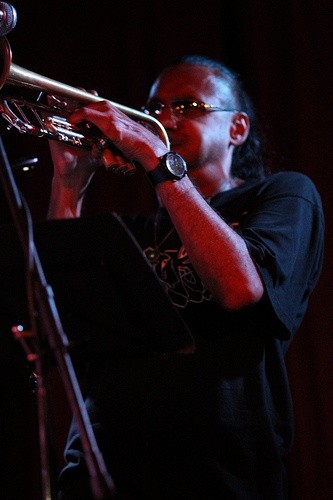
[0,35,171,176]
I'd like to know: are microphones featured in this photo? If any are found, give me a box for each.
[0,1,18,36]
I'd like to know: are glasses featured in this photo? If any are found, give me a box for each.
[141,99,240,119]
